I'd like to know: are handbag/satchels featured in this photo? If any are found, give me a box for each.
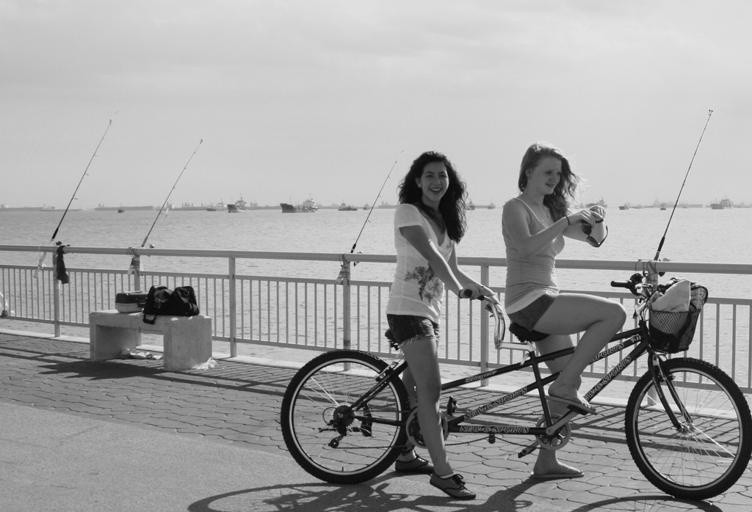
[643,277,708,352]
[141,285,200,326]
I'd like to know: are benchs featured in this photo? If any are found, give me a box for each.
[89,311,212,373]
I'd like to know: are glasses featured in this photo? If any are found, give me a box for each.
[581,219,609,248]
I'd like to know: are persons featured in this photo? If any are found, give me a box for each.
[499,141,630,480]
[381,150,499,500]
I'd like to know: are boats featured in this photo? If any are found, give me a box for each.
[339,203,357,211]
[228,203,247,214]
[281,200,318,213]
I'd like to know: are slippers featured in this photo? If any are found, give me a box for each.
[543,391,598,415]
[530,465,583,478]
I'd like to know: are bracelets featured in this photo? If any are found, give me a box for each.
[565,215,571,226]
[595,218,603,223]
[457,289,463,299]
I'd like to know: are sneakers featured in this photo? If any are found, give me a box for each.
[394,455,434,473]
[429,473,475,499]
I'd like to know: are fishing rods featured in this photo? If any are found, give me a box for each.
[654,109,711,261]
[32,118,112,278]
[334,160,395,286]
[126,138,202,276]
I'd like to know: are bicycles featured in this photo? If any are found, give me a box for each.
[280,273,751,499]
